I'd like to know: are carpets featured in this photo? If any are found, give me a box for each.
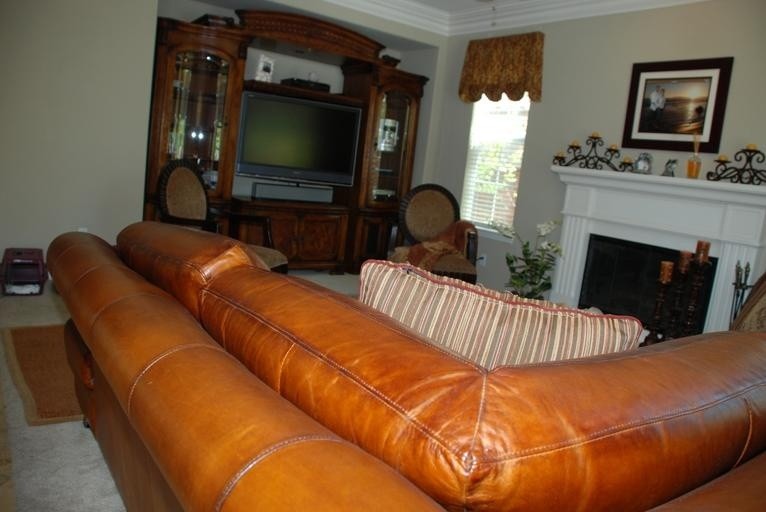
[0,325,84,426]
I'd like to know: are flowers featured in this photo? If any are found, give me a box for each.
[489,218,563,300]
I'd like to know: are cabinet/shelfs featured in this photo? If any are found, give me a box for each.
[142,9,430,274]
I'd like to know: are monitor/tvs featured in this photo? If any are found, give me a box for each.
[235,90,362,204]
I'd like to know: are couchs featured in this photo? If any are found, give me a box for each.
[47,222,766,512]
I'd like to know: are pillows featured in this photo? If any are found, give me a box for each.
[360,260,643,371]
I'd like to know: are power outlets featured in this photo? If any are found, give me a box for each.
[478,255,486,266]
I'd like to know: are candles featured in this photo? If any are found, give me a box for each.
[746,144,757,149]
[678,251,692,274]
[559,131,633,162]
[659,261,674,284]
[719,156,728,161]
[695,240,711,263]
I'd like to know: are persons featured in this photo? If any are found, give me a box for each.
[649,85,666,129]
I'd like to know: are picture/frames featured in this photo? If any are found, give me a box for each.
[621,56,734,154]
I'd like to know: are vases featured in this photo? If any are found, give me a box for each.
[687,154,702,179]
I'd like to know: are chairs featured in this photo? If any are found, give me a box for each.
[386,184,478,285]
